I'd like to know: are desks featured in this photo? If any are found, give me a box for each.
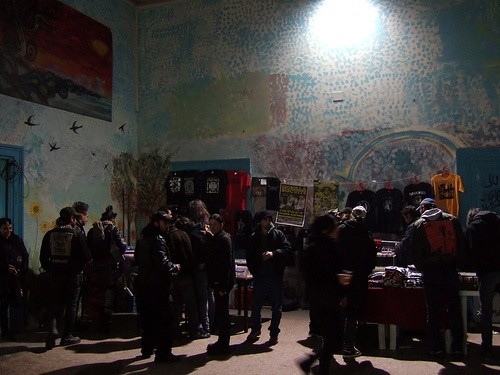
[366,287,484,358]
[105,269,253,334]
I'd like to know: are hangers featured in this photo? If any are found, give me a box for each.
[442,166,449,177]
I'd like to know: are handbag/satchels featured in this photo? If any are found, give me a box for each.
[110,225,125,264]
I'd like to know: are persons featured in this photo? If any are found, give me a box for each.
[295,205,377,358]
[39,201,127,349]
[464,208,500,355]
[401,204,419,226]
[244,210,291,345]
[135,199,235,361]
[0,217,29,334]
[395,198,464,358]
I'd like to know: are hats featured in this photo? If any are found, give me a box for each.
[256,211,271,222]
[151,210,173,223]
[60,206,82,218]
[416,197,435,211]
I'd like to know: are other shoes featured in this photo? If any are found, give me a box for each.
[300,359,312,374]
[267,335,278,345]
[59,333,81,346]
[142,353,151,359]
[154,350,185,364]
[45,328,59,349]
[343,345,362,357]
[247,330,261,343]
[206,339,230,355]
